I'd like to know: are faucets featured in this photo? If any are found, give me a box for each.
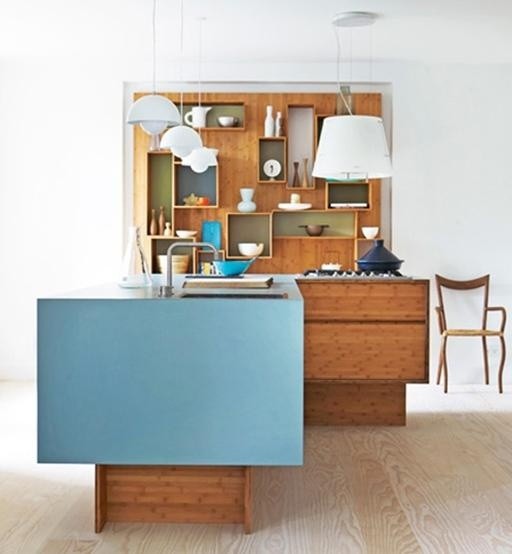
[167,242,219,292]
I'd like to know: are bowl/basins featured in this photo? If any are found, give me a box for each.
[237,242,264,256]
[211,258,254,277]
[156,252,190,275]
[361,226,380,240]
[297,223,329,237]
[217,115,239,126]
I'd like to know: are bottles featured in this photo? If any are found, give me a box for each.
[292,158,310,188]
[149,203,173,239]
[263,103,284,138]
[118,226,153,289]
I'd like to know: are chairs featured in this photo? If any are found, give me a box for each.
[435,274,506,393]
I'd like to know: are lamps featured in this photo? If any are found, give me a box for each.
[126,0,219,174]
[312,12,394,181]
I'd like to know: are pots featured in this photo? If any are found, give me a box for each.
[354,239,405,272]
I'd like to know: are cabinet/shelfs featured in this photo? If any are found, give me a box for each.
[133,93,381,273]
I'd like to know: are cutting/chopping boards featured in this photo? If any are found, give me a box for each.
[180,273,274,288]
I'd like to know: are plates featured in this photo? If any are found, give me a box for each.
[175,228,199,238]
[262,159,282,178]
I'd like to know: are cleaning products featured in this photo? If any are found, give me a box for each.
[265,105,275,138]
[275,112,283,136]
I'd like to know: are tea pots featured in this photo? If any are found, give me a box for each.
[183,106,212,129]
